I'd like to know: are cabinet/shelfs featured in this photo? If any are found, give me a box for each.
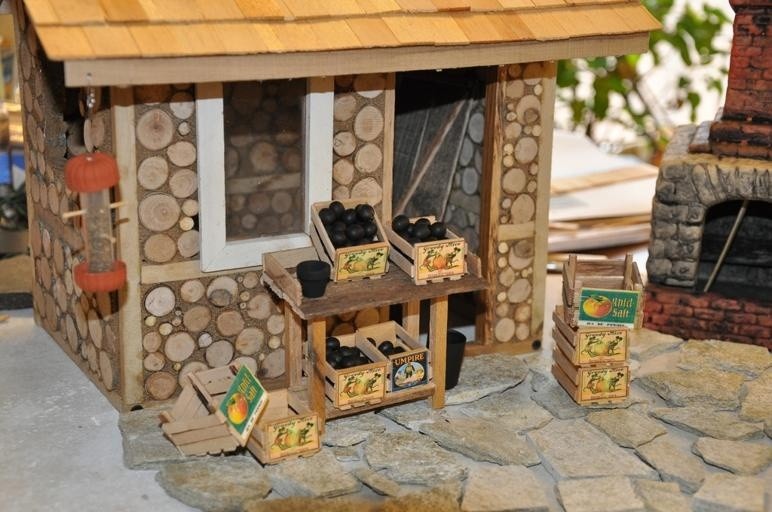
[262,243,490,437]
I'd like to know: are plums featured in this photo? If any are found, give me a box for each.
[367,337,406,356]
[324,337,369,369]
[392,215,448,243]
[319,200,378,249]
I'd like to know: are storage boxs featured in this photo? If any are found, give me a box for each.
[310,198,391,282]
[385,215,468,287]
[159,361,324,466]
[549,256,645,406]
[304,321,435,410]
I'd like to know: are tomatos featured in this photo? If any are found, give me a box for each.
[582,294,612,318]
[226,393,248,425]
